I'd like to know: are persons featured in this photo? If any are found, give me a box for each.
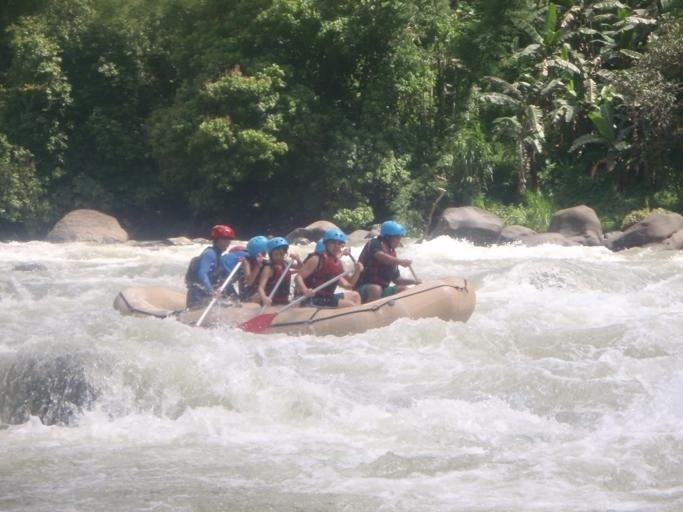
[182,221,410,309]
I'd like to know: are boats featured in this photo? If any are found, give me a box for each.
[108,272,477,335]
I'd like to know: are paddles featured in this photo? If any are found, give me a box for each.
[236,269,356,333]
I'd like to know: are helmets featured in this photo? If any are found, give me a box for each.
[247,236,269,257]
[380,220,407,238]
[323,229,345,244]
[210,224,235,240]
[265,236,289,257]
[315,238,327,255]
[229,246,246,253]
